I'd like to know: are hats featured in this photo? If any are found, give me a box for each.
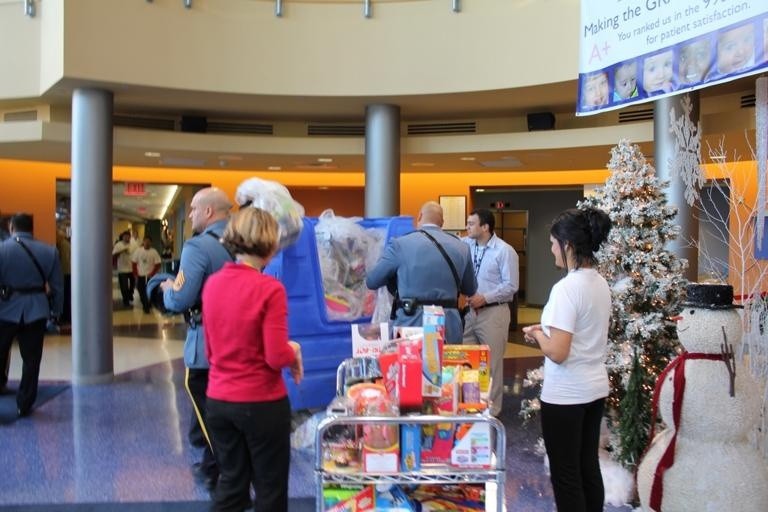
[146,271,181,319]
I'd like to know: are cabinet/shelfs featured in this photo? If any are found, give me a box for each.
[309,358,506,512]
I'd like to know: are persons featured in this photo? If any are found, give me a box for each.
[519,203,612,512]
[642,51,674,97]
[130,229,139,244]
[111,229,139,308]
[1,208,66,420]
[198,205,305,512]
[714,22,756,77]
[611,62,639,101]
[57,236,71,326]
[581,71,608,107]
[154,185,237,502]
[676,36,711,91]
[363,198,479,347]
[131,234,162,314]
[458,206,518,420]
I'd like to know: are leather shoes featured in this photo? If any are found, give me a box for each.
[191,461,218,491]
[16,407,33,418]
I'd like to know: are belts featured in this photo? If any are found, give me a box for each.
[416,298,458,308]
[477,301,507,308]
[11,287,47,295]
